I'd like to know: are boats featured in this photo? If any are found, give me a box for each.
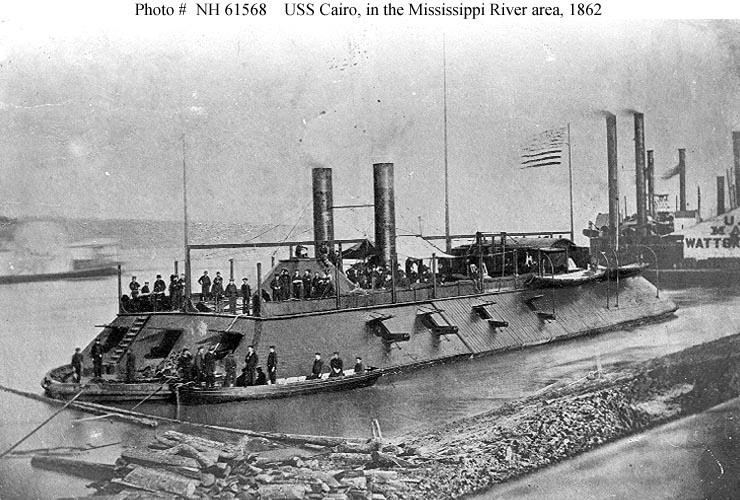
[582,109,740,292]
[162,363,383,404]
[39,43,681,403]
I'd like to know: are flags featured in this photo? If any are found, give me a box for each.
[518,124,567,170]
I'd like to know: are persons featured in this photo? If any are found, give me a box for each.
[67,242,540,387]
